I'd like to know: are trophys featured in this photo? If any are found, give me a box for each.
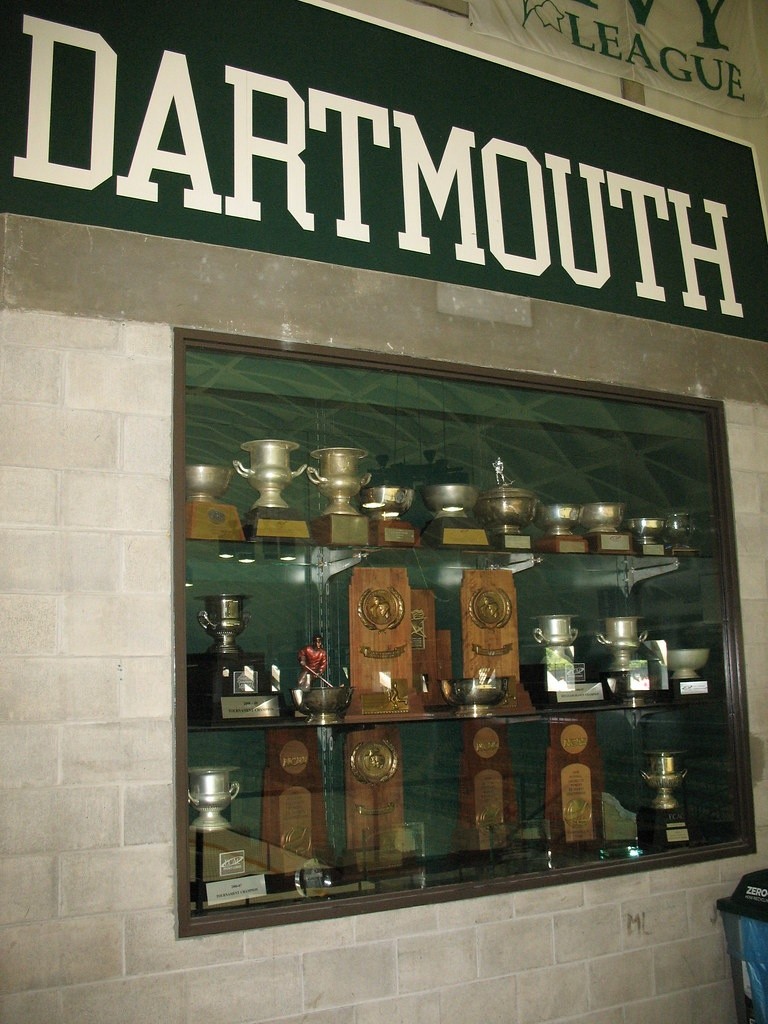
[184,440,715,877]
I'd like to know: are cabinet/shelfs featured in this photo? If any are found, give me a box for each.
[186,538,737,920]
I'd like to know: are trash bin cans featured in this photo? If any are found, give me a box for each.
[715,867,768,1024]
